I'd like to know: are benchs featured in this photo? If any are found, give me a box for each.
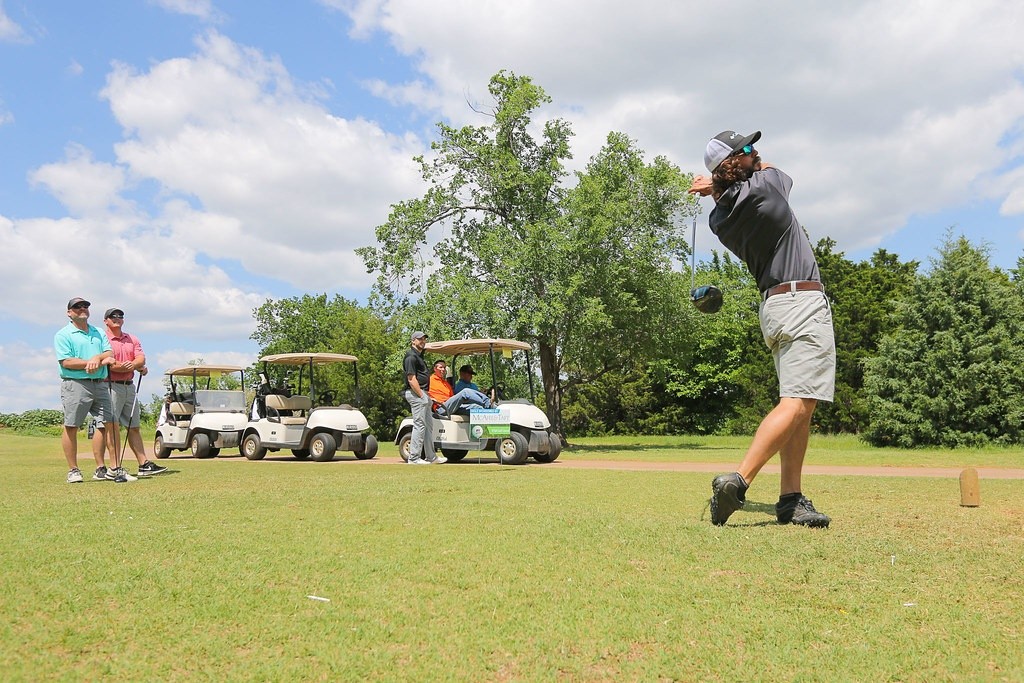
[265,394,312,425]
[444,414,470,423]
[169,402,194,428]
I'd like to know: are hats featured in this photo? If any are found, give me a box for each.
[411,331,428,340]
[104,308,124,320]
[68,297,91,310]
[458,365,477,376]
[704,130,762,172]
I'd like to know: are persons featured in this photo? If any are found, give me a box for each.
[55,297,139,483]
[428,360,498,416]
[93,307,167,481]
[686,129,836,529]
[402,330,448,465]
[454,365,488,396]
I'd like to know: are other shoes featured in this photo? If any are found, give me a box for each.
[485,388,495,409]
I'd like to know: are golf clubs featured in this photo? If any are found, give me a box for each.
[120,372,142,469]
[691,192,723,314]
[107,364,127,483]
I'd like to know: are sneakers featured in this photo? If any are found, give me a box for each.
[431,456,447,464]
[775,495,829,527]
[709,473,746,527]
[408,458,431,464]
[67,468,83,483]
[104,466,138,482]
[137,460,168,476]
[93,465,107,480]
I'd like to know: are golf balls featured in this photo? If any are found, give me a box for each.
[283,369,296,390]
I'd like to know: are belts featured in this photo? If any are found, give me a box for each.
[759,282,824,301]
[111,380,133,386]
[85,378,105,383]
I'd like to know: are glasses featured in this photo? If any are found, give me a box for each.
[732,144,754,159]
[106,313,123,319]
[71,303,90,309]
